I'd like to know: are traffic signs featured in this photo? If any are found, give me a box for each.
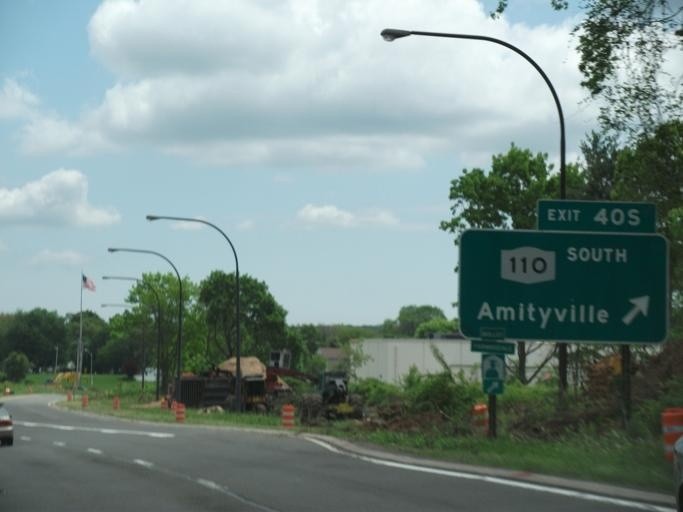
[534,196,656,233]
[457,228,671,349]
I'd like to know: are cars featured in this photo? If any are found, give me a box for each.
[0,403,15,446]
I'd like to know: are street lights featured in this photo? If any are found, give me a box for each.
[53,344,58,374]
[379,27,577,429]
[83,348,93,387]
[144,214,240,414]
[106,246,182,405]
[100,304,145,391]
[102,275,160,402]
